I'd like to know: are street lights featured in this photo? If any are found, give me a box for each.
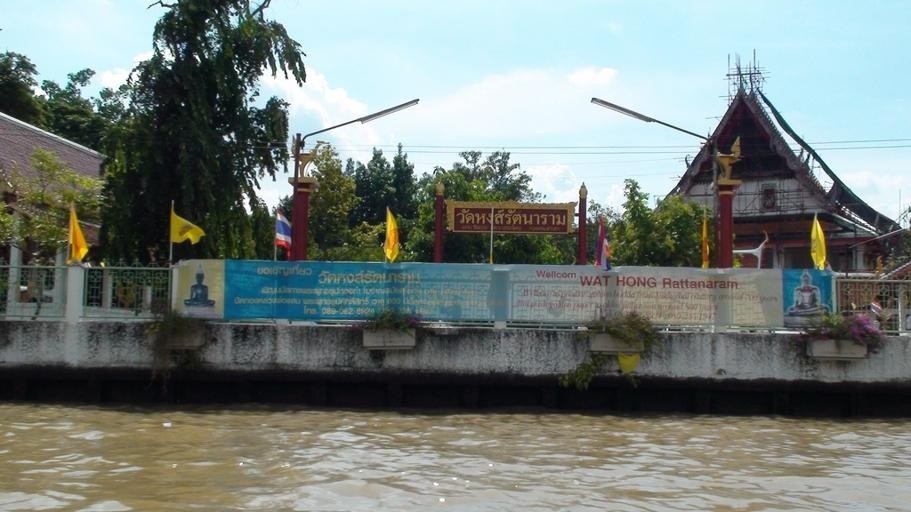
[291,99,420,261]
[591,97,720,268]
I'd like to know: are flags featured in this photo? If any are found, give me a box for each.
[169,205,206,246]
[810,216,826,271]
[273,209,294,261]
[382,208,400,264]
[594,214,611,271]
[65,203,89,266]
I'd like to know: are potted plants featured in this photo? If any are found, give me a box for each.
[561,312,665,394]
[147,307,215,389]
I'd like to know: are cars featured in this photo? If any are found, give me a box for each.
[841,272,911,333]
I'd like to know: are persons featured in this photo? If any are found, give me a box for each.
[788,274,822,313]
[183,271,215,310]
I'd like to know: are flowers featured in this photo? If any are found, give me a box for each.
[352,312,427,342]
[793,310,885,355]
